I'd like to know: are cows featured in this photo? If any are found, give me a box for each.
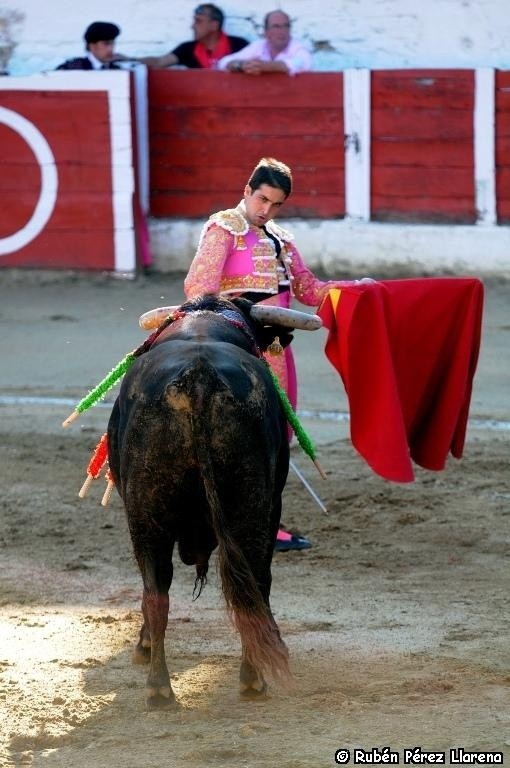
[106,290,324,712]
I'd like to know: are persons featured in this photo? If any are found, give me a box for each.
[108,2,252,70]
[52,20,149,72]
[219,10,314,79]
[180,158,383,553]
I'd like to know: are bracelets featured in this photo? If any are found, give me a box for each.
[233,60,245,73]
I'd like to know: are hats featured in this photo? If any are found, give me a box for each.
[84,22,119,43]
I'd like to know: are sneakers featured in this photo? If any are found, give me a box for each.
[275,531,312,553]
